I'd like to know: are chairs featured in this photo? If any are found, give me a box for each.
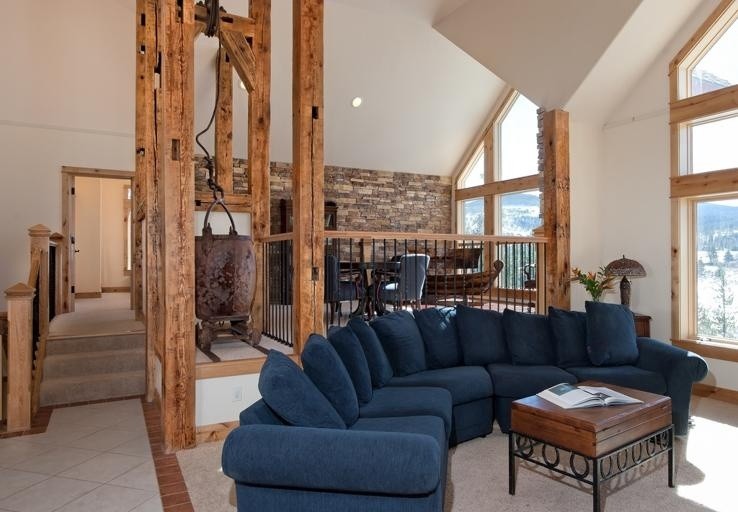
[324,242,431,317]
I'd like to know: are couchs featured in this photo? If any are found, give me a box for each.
[222,336,710,512]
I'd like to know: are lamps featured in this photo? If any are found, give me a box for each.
[605,253,647,306]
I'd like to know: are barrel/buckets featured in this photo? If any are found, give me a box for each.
[195,199,255,320]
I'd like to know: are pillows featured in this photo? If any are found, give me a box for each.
[327,324,372,405]
[369,312,425,377]
[348,317,393,389]
[455,303,510,367]
[257,349,347,430]
[547,305,586,366]
[300,333,359,424]
[502,307,559,366]
[414,308,462,369]
[582,300,638,367]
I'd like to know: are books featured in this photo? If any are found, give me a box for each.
[536,383,644,409]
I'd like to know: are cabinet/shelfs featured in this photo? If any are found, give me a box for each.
[633,312,651,337]
